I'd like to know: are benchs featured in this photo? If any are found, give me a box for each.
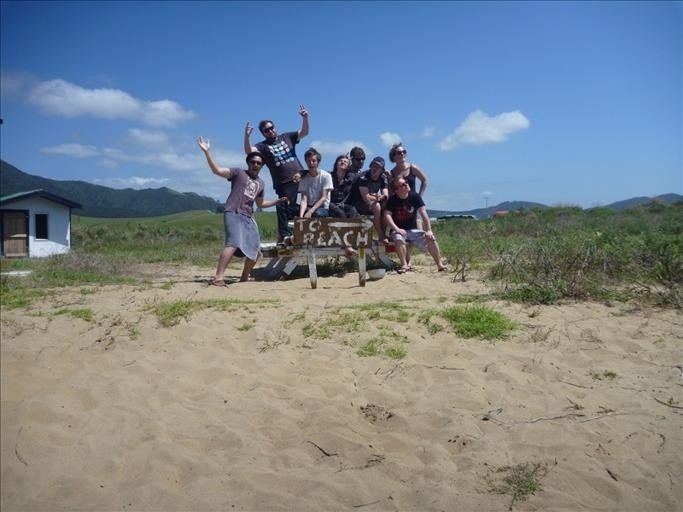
[262,217,395,289]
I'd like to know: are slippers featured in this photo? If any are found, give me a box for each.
[398,265,411,273]
[438,267,448,272]
[209,280,227,287]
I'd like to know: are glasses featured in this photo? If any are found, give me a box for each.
[251,160,261,165]
[354,157,364,162]
[399,181,409,189]
[394,149,405,155]
[263,126,274,134]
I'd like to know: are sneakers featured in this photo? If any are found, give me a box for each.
[283,235,295,245]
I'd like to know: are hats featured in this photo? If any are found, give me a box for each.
[246,151,264,166]
[370,157,384,171]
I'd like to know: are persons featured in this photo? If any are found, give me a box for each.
[349,156,391,246]
[382,174,448,275]
[345,146,366,179]
[388,143,428,196]
[193,133,288,287]
[296,147,333,219]
[243,104,311,243]
[291,153,360,217]
